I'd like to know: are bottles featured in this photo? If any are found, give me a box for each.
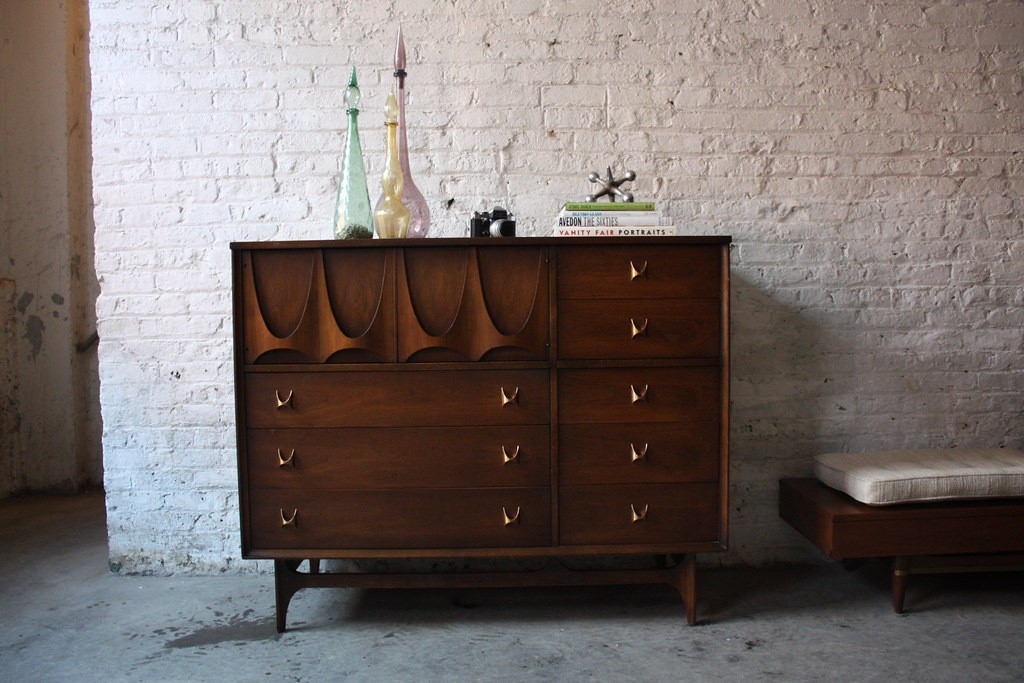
[333,22,430,239]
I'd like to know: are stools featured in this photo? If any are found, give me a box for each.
[779,449,1024,613]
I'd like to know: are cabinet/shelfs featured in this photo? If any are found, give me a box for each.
[230,234,734,634]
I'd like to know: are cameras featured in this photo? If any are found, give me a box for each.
[469,205,514,238]
